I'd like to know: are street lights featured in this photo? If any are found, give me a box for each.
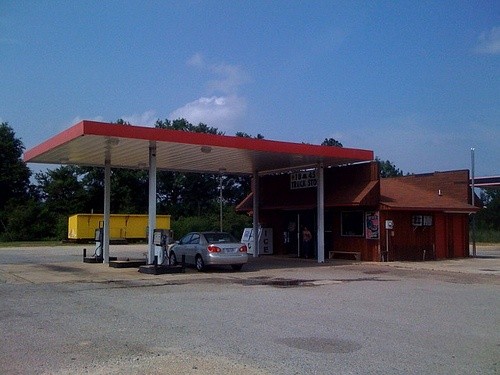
[470,147,476,258]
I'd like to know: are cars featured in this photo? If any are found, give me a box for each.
[169,232,249,272]
